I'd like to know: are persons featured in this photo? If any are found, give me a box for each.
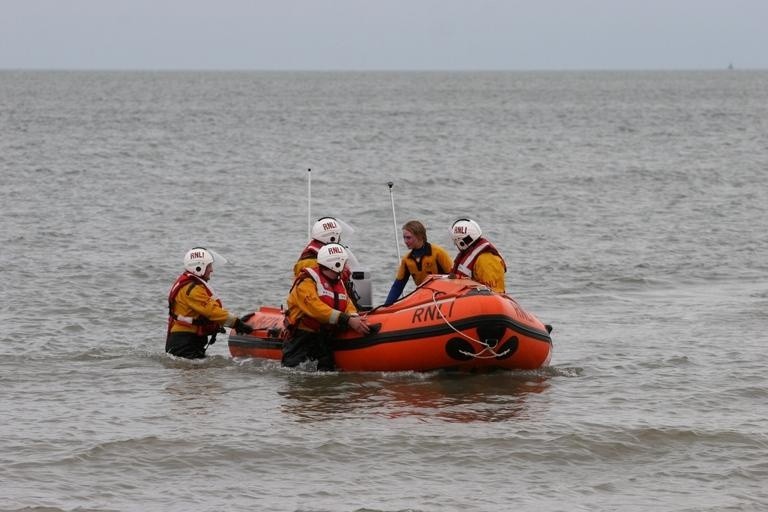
[289,215,361,312]
[163,246,253,360]
[448,216,507,294]
[279,241,371,371]
[382,220,455,308]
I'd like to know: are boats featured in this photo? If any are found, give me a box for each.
[230,272,553,376]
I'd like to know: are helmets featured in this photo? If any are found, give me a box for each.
[312,216,353,244]
[316,243,360,273]
[449,218,483,251]
[183,247,226,277]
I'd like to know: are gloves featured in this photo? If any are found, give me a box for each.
[234,313,255,335]
